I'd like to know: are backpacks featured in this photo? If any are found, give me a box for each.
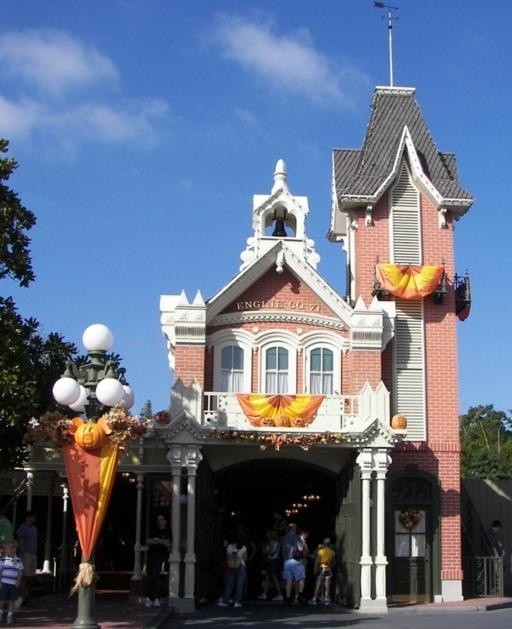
[226,552,242,570]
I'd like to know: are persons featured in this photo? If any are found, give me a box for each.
[1,540,24,627]
[16,509,39,604]
[1,510,25,613]
[144,513,172,607]
[215,521,337,607]
[482,517,507,561]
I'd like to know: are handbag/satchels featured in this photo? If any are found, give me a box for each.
[292,544,305,561]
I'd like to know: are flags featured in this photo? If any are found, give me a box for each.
[62,438,121,586]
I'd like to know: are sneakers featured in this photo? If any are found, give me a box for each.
[153,598,160,607]
[144,597,152,607]
[216,597,233,608]
[15,596,24,610]
[285,595,333,608]
[257,593,267,599]
[0,613,4,622]
[233,601,243,608]
[7,614,14,624]
[272,594,284,601]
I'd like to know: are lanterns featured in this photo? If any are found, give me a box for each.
[262,416,273,427]
[274,415,290,427]
[391,415,407,429]
[155,410,170,425]
[290,417,305,427]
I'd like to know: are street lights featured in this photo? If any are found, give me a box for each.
[48,322,135,629]
